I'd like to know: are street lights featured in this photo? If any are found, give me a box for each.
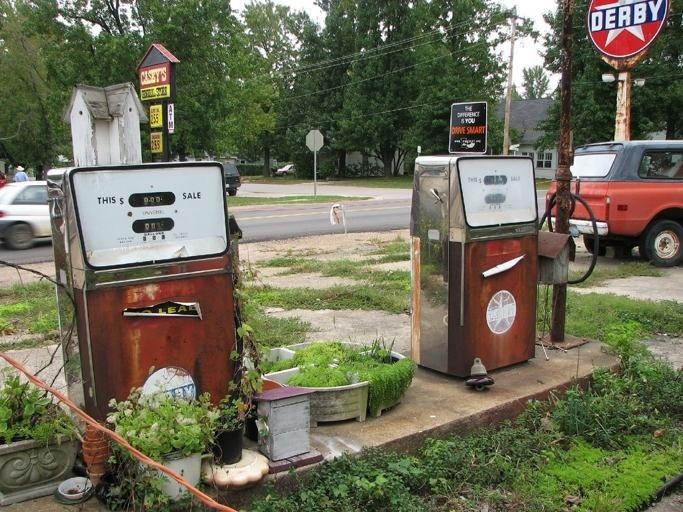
[600,72,647,143]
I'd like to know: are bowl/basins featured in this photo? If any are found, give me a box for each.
[58,477,92,499]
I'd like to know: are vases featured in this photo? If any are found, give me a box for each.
[81,423,110,488]
[138,452,201,502]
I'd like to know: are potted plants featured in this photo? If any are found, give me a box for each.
[255,341,415,428]
[0,374,78,507]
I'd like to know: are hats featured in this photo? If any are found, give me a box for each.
[16,165,24,171]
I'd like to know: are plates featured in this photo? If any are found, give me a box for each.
[53,483,95,504]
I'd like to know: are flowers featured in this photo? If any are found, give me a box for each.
[106,295,269,507]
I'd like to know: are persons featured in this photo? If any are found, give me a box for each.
[15,166,28,182]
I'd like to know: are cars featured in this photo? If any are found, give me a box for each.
[275,165,296,177]
[0,181,53,250]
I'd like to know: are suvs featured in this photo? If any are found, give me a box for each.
[222,163,242,196]
[544,138,682,268]
